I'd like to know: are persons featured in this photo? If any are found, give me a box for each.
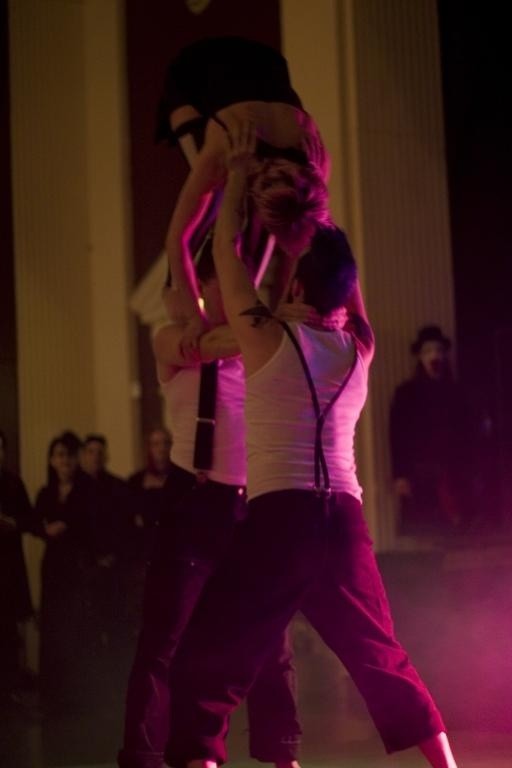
[153,38,330,373]
[388,325,470,540]
[166,120,458,768]
[118,231,350,768]
[0,425,197,682]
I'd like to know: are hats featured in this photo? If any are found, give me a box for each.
[410,326,450,354]
